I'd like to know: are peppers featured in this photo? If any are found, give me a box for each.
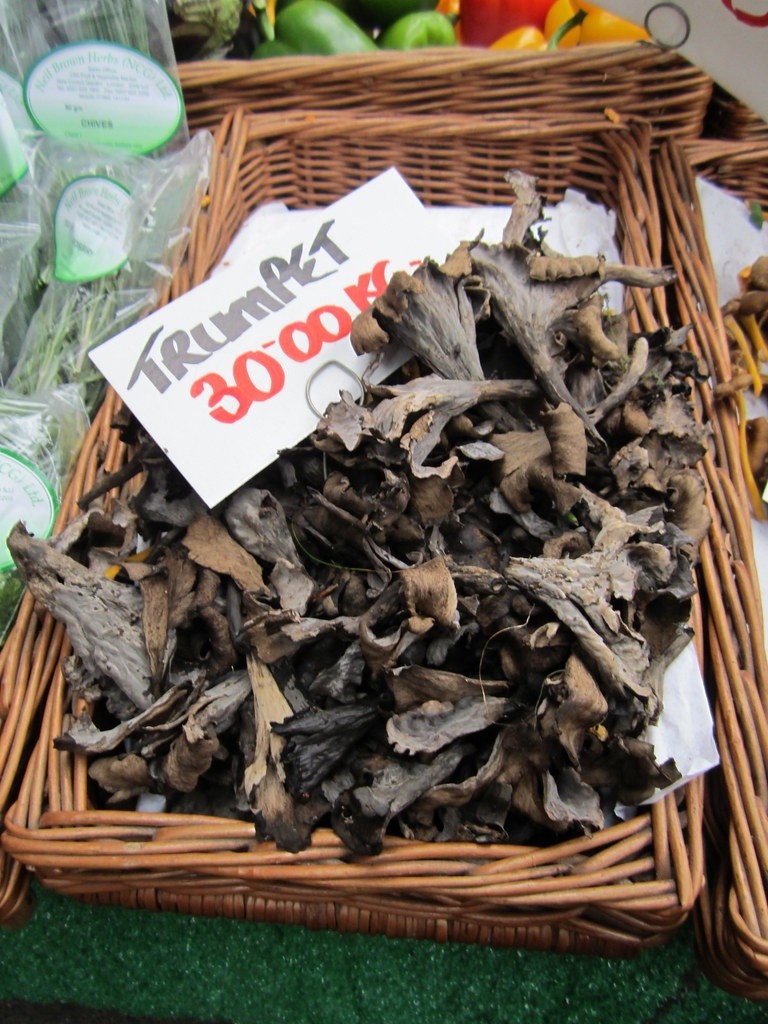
[248,0,651,62]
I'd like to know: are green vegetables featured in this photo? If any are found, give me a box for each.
[0,0,245,649]
